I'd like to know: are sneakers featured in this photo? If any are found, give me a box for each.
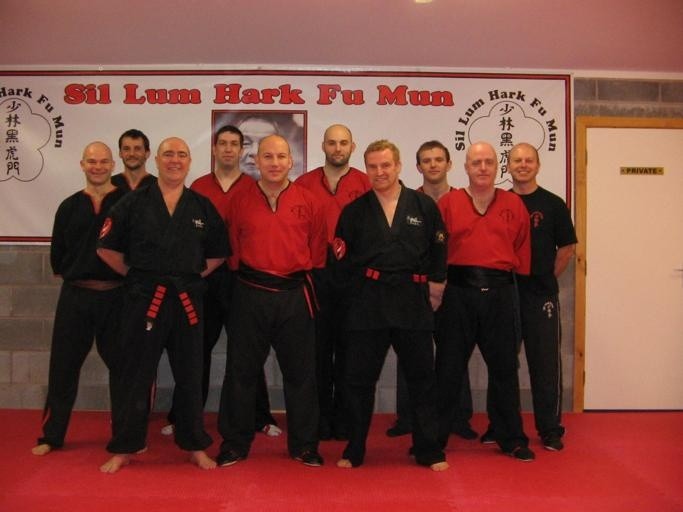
[543,436,563,451]
[508,443,534,462]
[217,448,241,467]
[296,450,323,467]
[480,430,497,444]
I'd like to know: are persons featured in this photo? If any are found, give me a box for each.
[238,114,281,179]
[479,141,579,456]
[409,141,536,463]
[330,137,452,473]
[290,123,373,441]
[28,139,150,456]
[94,137,234,474]
[158,124,284,440]
[213,135,329,468]
[384,137,480,441]
[107,128,157,198]
